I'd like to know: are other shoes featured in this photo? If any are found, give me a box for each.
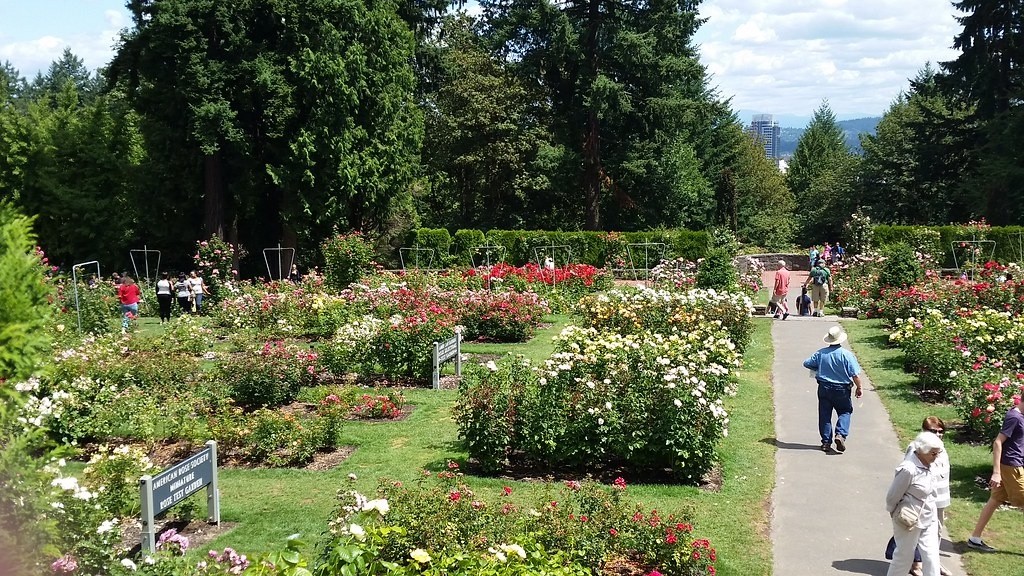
[813,312,817,316]
[939,562,952,576]
[783,312,789,320]
[967,538,996,552]
[910,565,923,576]
[834,434,845,452]
[819,313,825,317]
[774,314,780,318]
[821,443,830,452]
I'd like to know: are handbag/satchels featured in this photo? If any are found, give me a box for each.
[899,506,919,526]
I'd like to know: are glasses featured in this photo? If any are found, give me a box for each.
[926,428,945,434]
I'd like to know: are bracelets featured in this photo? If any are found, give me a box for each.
[804,285,806,286]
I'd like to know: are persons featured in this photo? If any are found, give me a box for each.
[803,327,863,451]
[120,276,140,334]
[87,274,97,284]
[765,297,777,314]
[543,252,553,270]
[796,288,810,316]
[289,264,299,281]
[111,273,121,284]
[772,260,791,320]
[809,241,845,267]
[156,271,174,326]
[886,431,944,576]
[968,391,1024,551]
[174,272,193,318]
[904,416,952,576]
[804,260,833,317]
[187,270,211,314]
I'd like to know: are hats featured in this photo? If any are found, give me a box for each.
[818,260,826,264]
[823,326,847,345]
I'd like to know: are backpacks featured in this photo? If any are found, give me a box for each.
[814,266,827,285]
